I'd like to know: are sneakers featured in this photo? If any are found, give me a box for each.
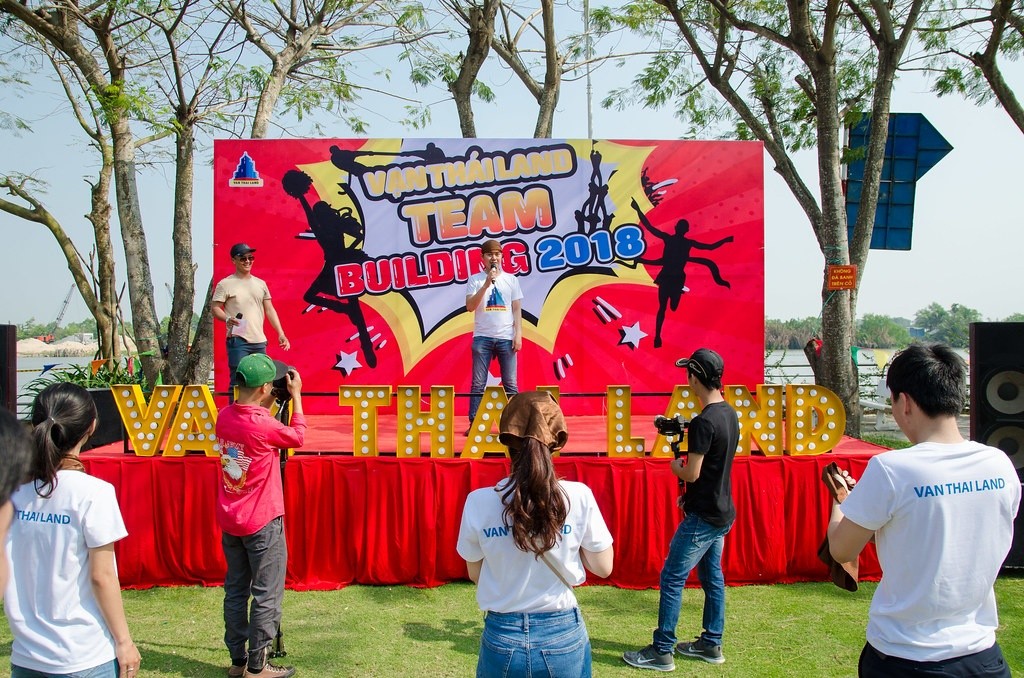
[228,664,246,678]
[623,644,675,672]
[676,636,726,664]
[246,660,295,678]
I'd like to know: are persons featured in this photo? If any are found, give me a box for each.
[456,390,614,678]
[212,243,290,405]
[623,347,740,672]
[0,384,141,678]
[827,341,1022,678]
[464,241,523,437]
[215,354,306,678]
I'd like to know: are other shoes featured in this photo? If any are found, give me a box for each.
[464,424,472,436]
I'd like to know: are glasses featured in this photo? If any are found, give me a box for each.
[235,256,255,262]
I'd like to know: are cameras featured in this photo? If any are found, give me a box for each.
[272,372,294,388]
[651,415,690,433]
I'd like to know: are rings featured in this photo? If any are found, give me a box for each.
[128,668,134,671]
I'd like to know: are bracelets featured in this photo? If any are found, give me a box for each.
[226,317,231,323]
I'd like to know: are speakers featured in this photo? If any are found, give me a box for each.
[0,324,18,418]
[968,321,1024,574]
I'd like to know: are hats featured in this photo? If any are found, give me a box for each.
[675,348,724,380]
[482,240,503,254]
[231,244,256,258]
[236,353,288,388]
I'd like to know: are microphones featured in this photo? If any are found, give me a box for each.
[491,264,497,284]
[228,313,243,336]
